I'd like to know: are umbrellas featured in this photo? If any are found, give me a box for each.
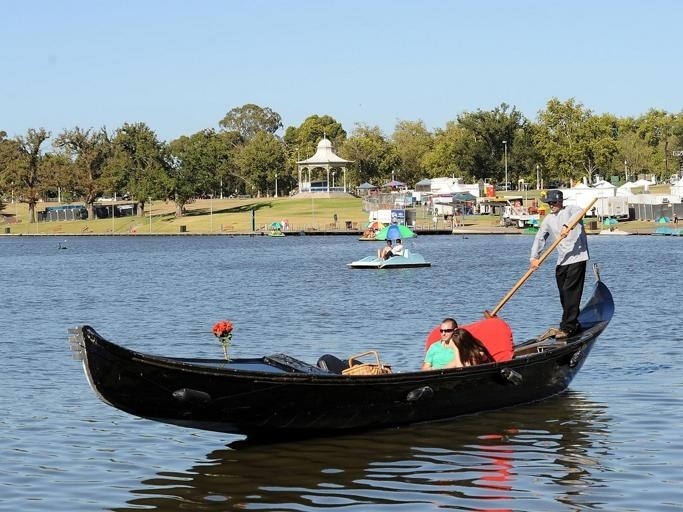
[356,182,378,188]
[364,222,385,229]
[528,218,538,230]
[373,224,417,245]
[656,216,670,232]
[271,223,283,232]
[602,219,618,226]
[384,181,404,186]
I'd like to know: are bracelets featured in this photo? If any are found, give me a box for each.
[562,224,568,228]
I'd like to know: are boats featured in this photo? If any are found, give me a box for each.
[601,228,627,234]
[347,249,430,267]
[359,233,375,240]
[67,265,615,438]
[269,231,285,236]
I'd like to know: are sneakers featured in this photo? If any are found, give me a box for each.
[554,324,582,340]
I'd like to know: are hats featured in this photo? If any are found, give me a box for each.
[538,189,568,204]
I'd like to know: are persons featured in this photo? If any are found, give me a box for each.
[530,189,590,338]
[422,318,496,371]
[433,207,439,229]
[280,216,289,229]
[453,212,465,228]
[673,214,678,230]
[362,228,381,238]
[378,239,404,261]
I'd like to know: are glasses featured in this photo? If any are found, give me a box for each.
[547,200,558,205]
[439,328,454,332]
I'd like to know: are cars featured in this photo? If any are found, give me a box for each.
[498,181,564,189]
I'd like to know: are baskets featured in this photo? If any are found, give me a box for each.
[340,350,392,375]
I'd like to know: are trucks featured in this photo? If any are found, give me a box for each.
[370,210,405,226]
[502,207,539,228]
[427,196,458,215]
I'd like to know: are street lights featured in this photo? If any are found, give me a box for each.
[502,141,507,192]
[625,160,627,181]
[536,165,539,189]
